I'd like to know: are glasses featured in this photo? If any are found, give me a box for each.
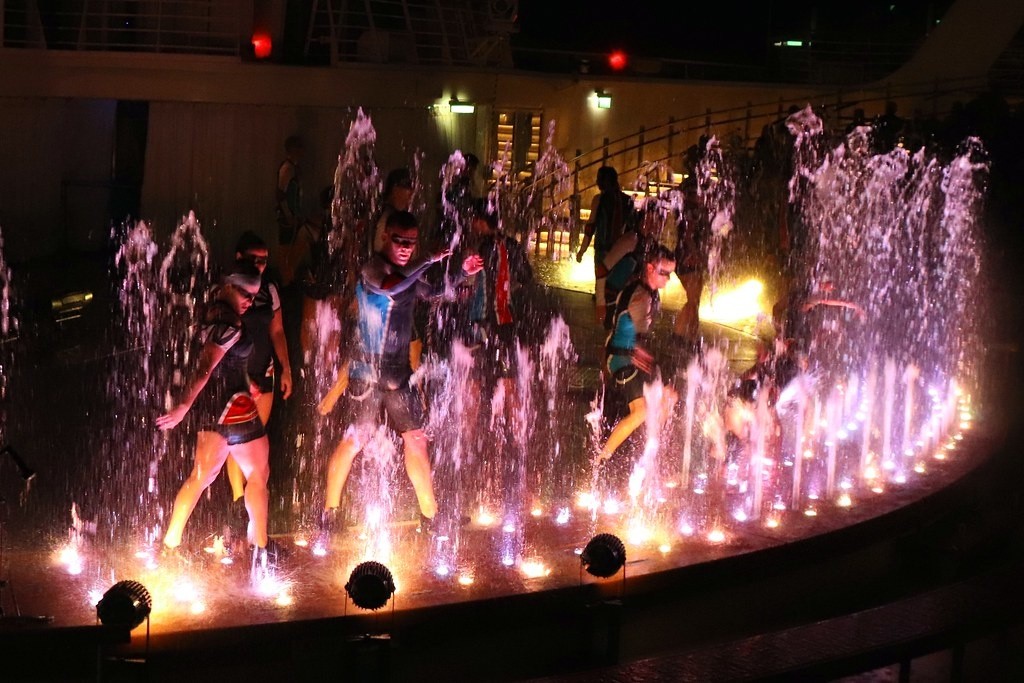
[232,283,258,301]
[657,267,672,276]
[242,252,268,265]
[389,233,420,246]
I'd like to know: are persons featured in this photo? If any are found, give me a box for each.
[270,133,307,282]
[147,262,291,579]
[576,164,642,323]
[206,229,297,533]
[452,194,536,464]
[299,185,357,382]
[592,205,678,377]
[436,151,502,264]
[678,79,1022,485]
[313,212,487,544]
[339,167,473,439]
[586,243,686,478]
